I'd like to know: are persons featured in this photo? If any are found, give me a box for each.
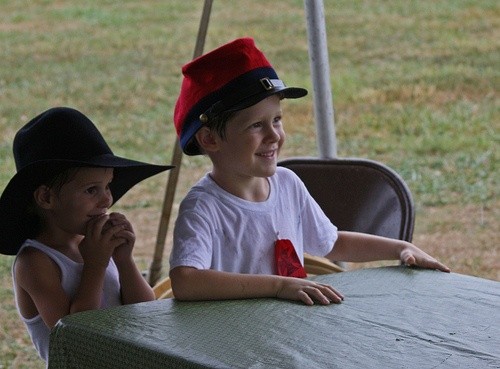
[169,37,450,305]
[11,107,156,364]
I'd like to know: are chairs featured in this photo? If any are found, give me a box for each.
[277,156,415,243]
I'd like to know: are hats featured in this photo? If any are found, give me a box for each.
[0,107,176,256]
[174,37,308,157]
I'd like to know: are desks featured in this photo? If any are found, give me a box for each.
[48,265,499,369]
